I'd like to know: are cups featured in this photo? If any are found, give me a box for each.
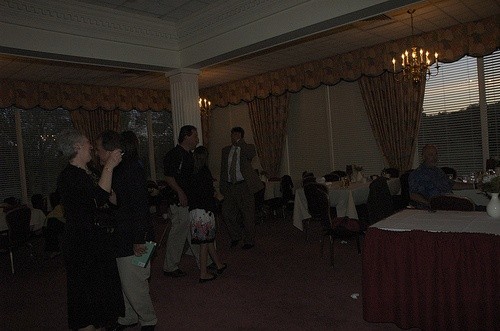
[448,169,495,183]
[315,169,390,188]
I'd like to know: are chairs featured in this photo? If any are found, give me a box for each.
[0,159,500,276]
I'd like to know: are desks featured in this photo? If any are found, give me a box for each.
[292,177,401,230]
[212,180,282,202]
[363,208,500,331]
[452,183,490,206]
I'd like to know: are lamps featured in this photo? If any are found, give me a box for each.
[199,98,212,120]
[391,9,439,83]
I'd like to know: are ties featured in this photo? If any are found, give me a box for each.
[229,148,238,185]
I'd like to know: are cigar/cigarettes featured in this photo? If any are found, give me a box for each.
[121,152,124,156]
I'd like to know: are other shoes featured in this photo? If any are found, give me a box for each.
[218,263,227,274]
[164,269,185,277]
[242,244,253,250]
[116,322,138,331]
[199,273,216,283]
[141,324,154,331]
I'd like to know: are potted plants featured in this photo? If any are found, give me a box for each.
[480,176,500,220]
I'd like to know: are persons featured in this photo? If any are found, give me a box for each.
[4,125,231,331]
[408,144,455,212]
[220,126,265,250]
